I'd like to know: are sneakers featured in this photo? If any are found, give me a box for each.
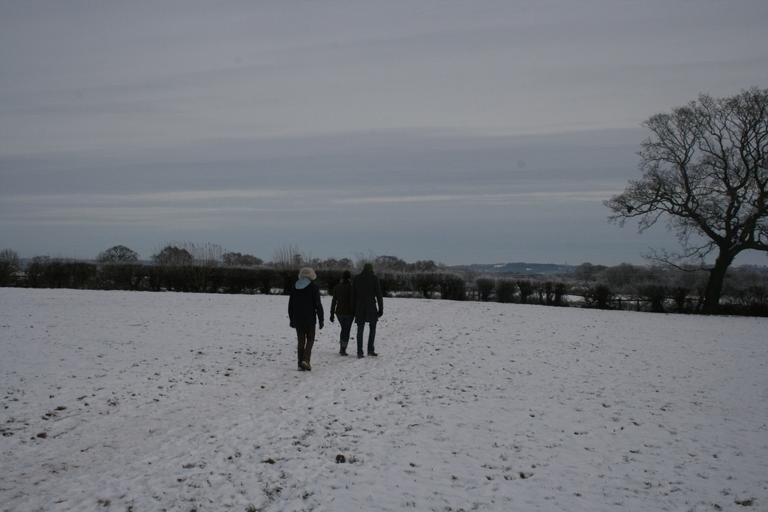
[367,351,378,358]
[301,358,311,371]
[298,362,305,371]
[338,350,348,356]
[356,353,364,359]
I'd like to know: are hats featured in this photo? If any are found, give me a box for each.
[342,270,351,279]
[298,267,317,281]
[364,263,374,273]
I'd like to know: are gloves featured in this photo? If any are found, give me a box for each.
[290,321,297,328]
[378,308,383,317]
[319,320,325,329]
[329,313,335,322]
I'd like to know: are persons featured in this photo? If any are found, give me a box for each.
[350,263,383,358]
[288,267,324,371]
[330,270,353,356]
